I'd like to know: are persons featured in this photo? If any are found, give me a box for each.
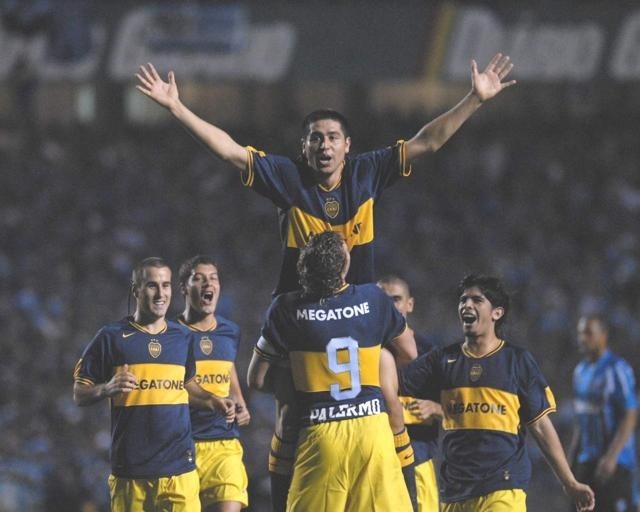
[396,270,596,512]
[374,273,445,511]
[245,229,419,510]
[166,254,252,511]
[563,311,639,510]
[132,52,519,510]
[71,254,236,511]
